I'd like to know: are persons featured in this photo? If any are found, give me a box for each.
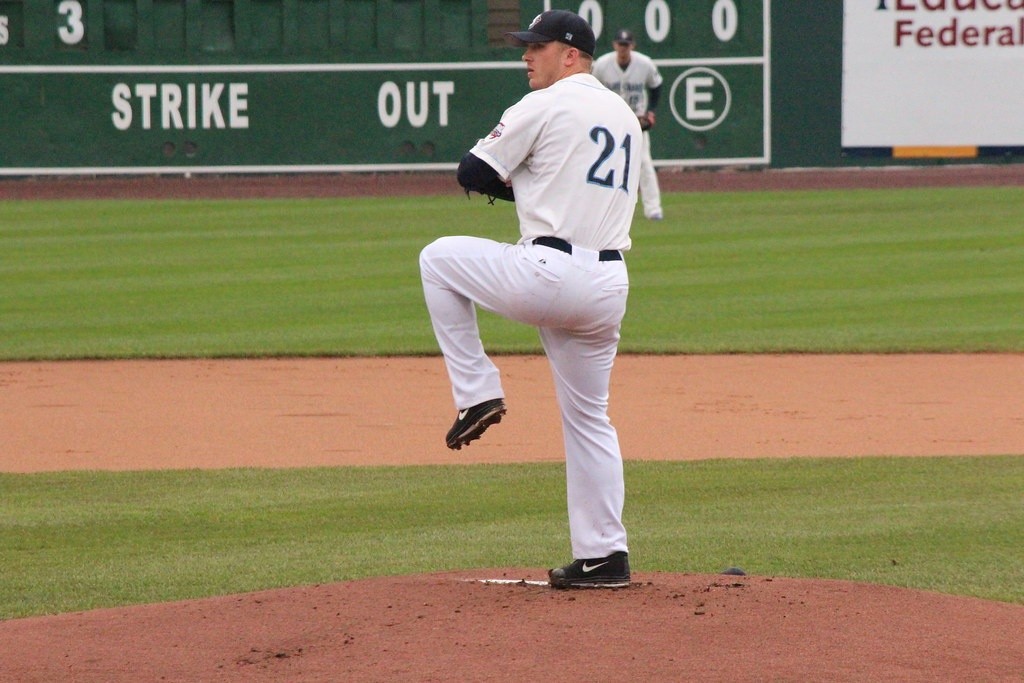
[419,10,643,586]
[593,29,664,219]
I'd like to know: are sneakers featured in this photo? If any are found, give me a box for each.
[446,398,508,450]
[549,551,630,588]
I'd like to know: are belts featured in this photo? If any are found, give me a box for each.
[532,236,622,261]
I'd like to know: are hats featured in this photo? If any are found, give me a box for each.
[504,10,595,56]
[613,29,633,44]
